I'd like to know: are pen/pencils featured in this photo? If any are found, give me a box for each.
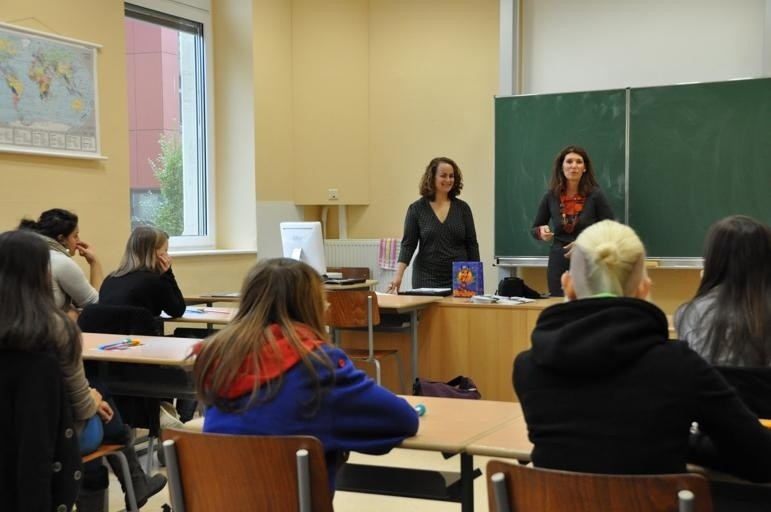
[414,405,425,415]
[100,339,131,349]
[104,340,139,350]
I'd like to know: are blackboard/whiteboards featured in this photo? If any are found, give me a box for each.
[493,76,771,270]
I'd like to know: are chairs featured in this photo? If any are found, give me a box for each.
[486,459,711,512]
[160,431,334,512]
[1,350,139,511]
[77,305,176,476]
[322,290,405,394]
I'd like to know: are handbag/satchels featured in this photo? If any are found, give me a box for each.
[411,372,482,401]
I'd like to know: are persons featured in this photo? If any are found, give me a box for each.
[510,217,770,511]
[0,229,168,512]
[184,258,419,505]
[99,225,198,423]
[530,145,616,297]
[383,156,481,297]
[673,214,771,366]
[17,208,103,323]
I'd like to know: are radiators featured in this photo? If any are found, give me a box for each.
[324,238,420,293]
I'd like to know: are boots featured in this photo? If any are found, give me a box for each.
[108,444,167,504]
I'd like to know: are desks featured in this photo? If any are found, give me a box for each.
[184,394,534,511]
[466,419,771,512]
[75,333,204,416]
[331,291,574,402]
[160,306,240,338]
[184,292,442,394]
[184,294,241,307]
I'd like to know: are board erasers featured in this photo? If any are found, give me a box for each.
[642,261,659,271]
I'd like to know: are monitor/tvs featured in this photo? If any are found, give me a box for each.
[279,220,327,277]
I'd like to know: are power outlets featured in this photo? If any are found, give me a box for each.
[329,189,339,200]
